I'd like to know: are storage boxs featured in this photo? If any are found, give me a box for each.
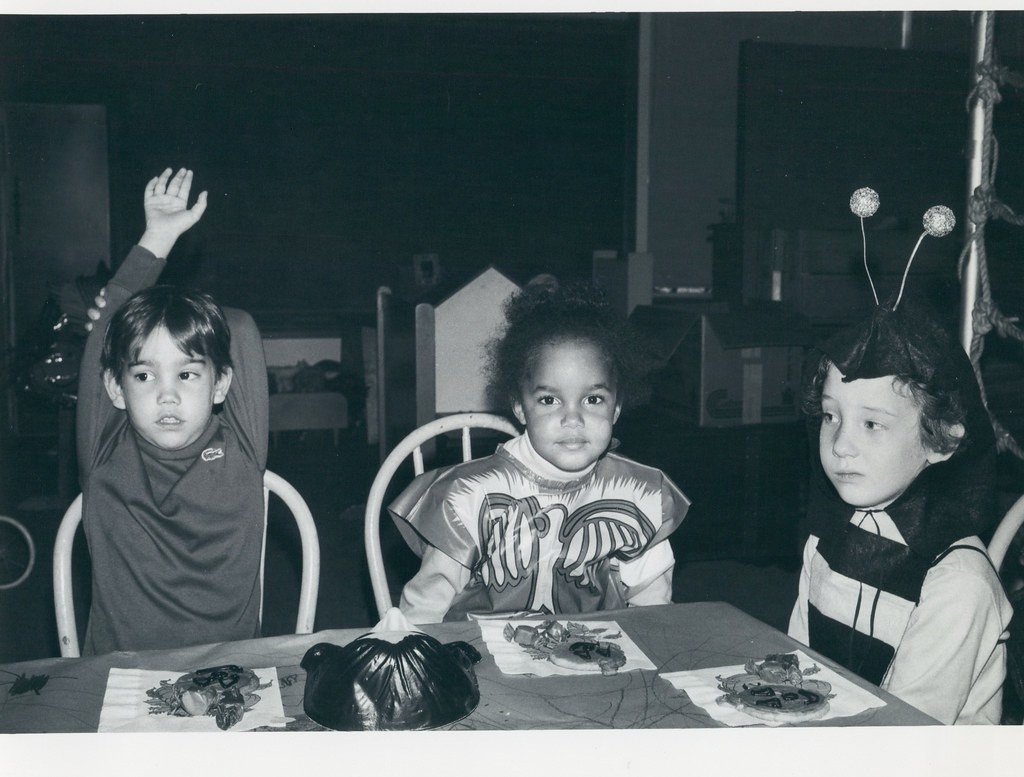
[618,303,818,428]
[759,222,943,343]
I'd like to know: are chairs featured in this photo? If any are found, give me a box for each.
[50,469,321,658]
[363,410,521,625]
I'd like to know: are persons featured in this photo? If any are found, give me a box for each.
[786,294,1014,727]
[75,168,270,657]
[385,278,692,624]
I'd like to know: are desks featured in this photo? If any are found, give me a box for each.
[0,600,946,733]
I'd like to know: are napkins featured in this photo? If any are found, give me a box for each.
[658,648,888,727]
[97,667,285,732]
[477,619,657,678]
